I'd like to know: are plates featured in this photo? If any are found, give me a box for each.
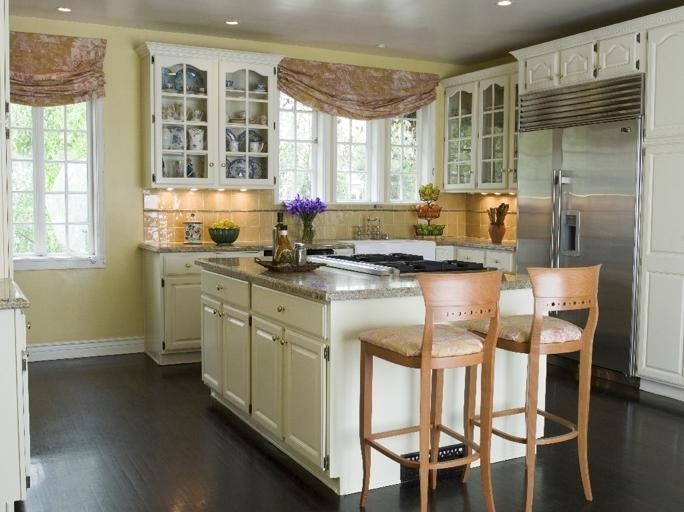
[225,129,264,152]
[173,69,203,94]
[226,159,264,179]
[225,87,234,90]
[160,68,173,92]
[230,117,254,125]
[253,89,265,92]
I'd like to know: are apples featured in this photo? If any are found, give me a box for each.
[416,203,442,219]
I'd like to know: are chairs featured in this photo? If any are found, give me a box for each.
[357,268,501,512]
[465,264,603,509]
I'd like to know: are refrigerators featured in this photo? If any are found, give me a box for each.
[514,72,644,390]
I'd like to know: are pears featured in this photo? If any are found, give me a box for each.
[415,223,447,236]
[418,182,440,201]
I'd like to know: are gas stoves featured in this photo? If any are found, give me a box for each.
[304,252,493,277]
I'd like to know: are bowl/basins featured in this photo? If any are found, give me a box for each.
[207,228,240,243]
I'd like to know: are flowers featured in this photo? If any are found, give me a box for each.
[283,193,328,214]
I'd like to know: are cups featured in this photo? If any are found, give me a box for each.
[226,80,233,86]
[256,82,264,88]
[192,111,203,121]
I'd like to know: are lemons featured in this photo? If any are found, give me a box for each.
[210,218,240,229]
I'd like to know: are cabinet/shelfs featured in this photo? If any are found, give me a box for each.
[455,244,519,270]
[139,242,263,367]
[440,58,519,193]
[2,279,33,512]
[251,272,338,480]
[508,14,647,92]
[636,3,684,411]
[134,39,282,189]
[199,261,251,418]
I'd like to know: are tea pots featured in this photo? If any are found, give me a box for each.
[164,125,207,153]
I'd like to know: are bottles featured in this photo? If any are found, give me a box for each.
[293,242,306,266]
[272,211,284,253]
[271,225,295,265]
[259,113,266,124]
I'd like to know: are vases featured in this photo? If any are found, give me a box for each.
[299,212,316,242]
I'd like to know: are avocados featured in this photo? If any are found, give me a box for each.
[277,249,293,266]
